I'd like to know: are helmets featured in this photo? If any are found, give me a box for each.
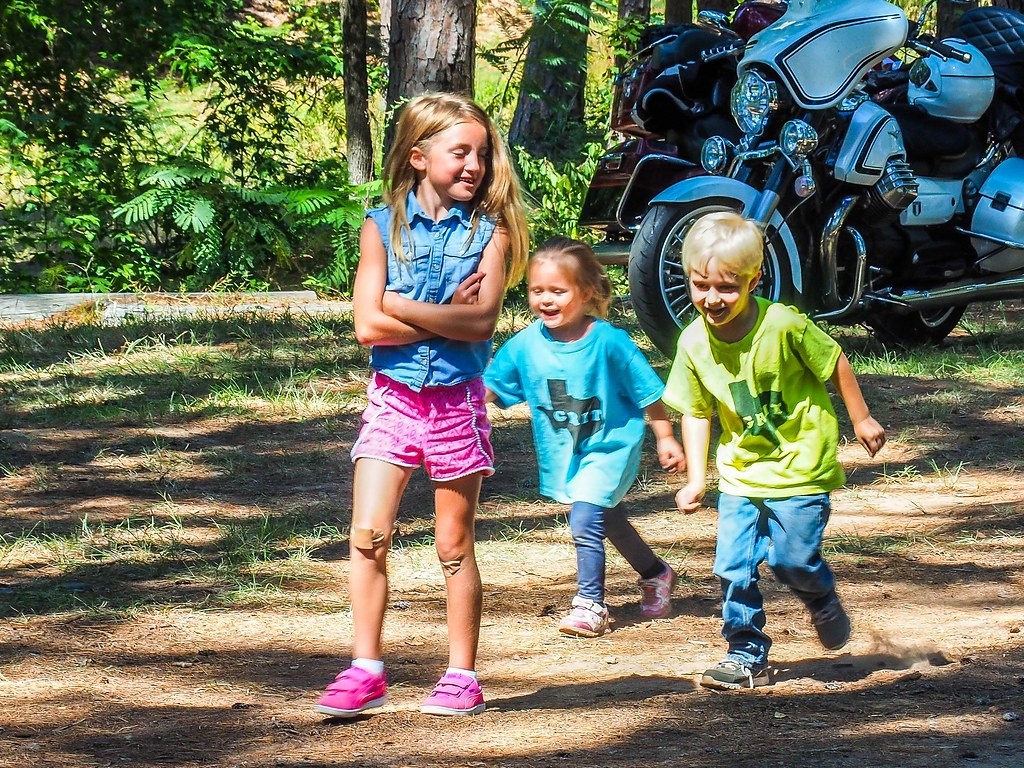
[907,37,995,124]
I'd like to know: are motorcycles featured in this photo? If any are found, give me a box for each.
[574,0,1023,367]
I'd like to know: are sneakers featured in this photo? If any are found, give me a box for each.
[421,673,486,717]
[559,596,612,637]
[810,598,851,650]
[638,558,677,620]
[313,667,388,718]
[701,659,770,690]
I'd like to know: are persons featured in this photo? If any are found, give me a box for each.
[310,91,529,718]
[483,235,686,642]
[661,211,886,689]
